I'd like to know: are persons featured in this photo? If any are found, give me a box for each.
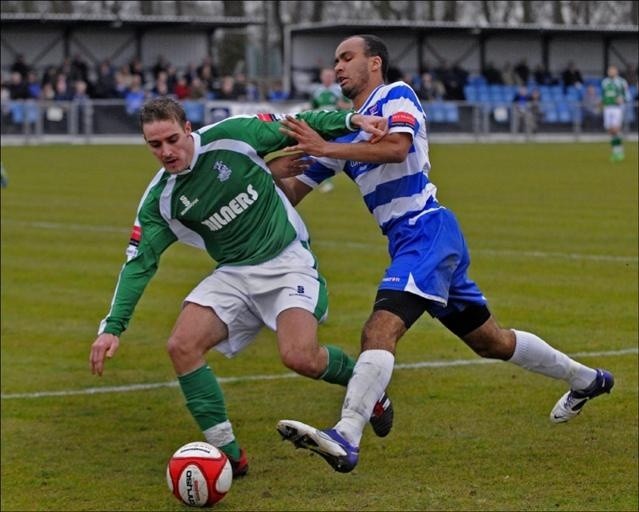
[265,34,614,473]
[601,66,631,162]
[0,53,639,133]
[90,98,394,479]
[301,66,354,193]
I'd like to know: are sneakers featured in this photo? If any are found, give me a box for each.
[610,152,625,163]
[276,419,359,473]
[550,368,614,424]
[368,390,393,437]
[225,448,249,478]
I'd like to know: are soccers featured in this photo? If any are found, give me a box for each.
[166,442,233,507]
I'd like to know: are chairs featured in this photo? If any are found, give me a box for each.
[424,78,637,125]
